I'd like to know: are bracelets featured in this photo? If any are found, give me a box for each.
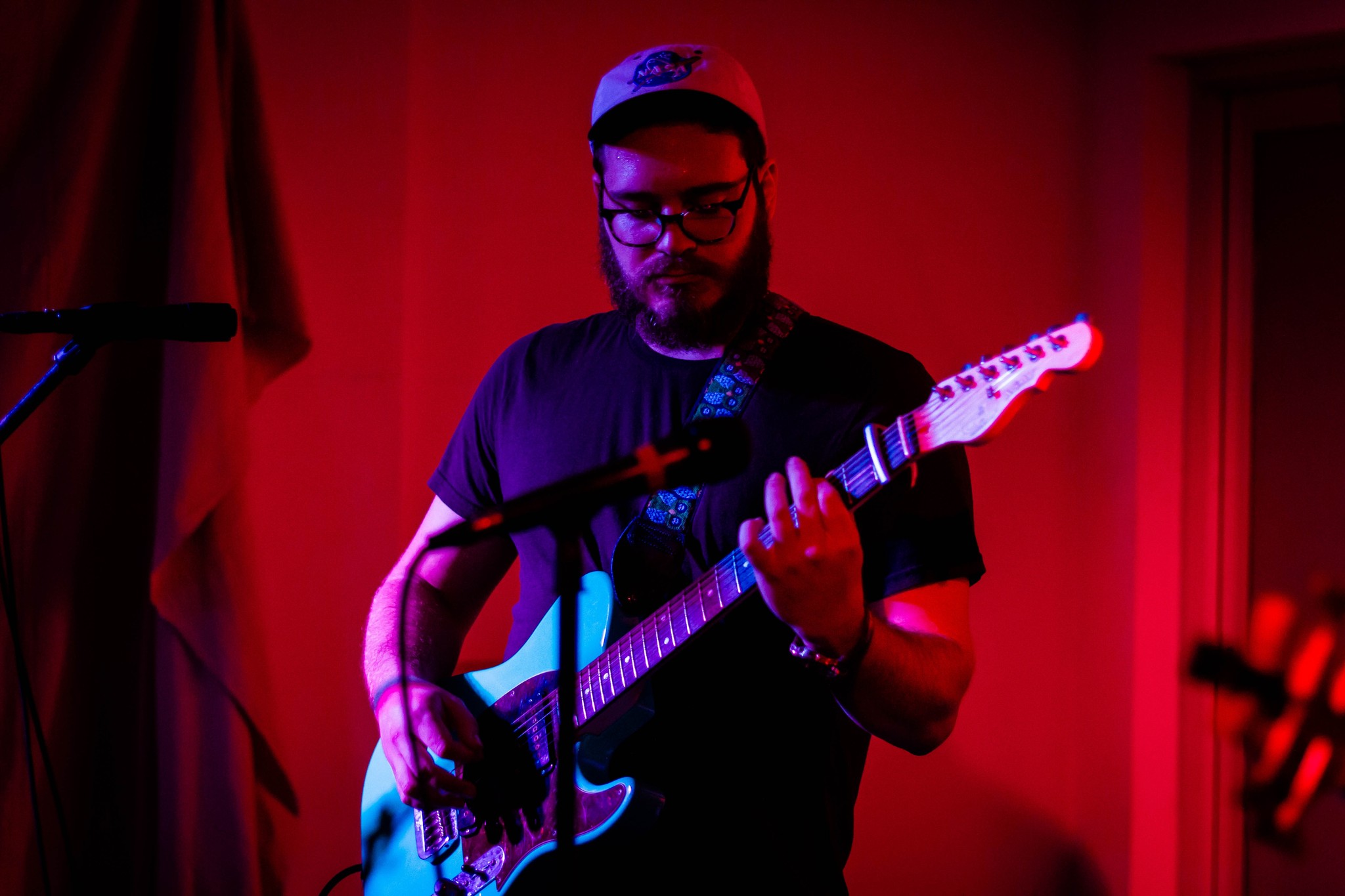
[373,675,429,710]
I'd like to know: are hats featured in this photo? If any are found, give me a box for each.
[588,46,768,163]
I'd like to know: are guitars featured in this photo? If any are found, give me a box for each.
[360,313,1103,896]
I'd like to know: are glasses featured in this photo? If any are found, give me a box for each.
[597,152,752,247]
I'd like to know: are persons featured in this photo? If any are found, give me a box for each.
[363,39,985,896]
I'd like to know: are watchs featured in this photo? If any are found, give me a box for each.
[789,608,874,679]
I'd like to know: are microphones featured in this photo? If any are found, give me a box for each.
[0,303,239,344]
[428,415,752,549]
